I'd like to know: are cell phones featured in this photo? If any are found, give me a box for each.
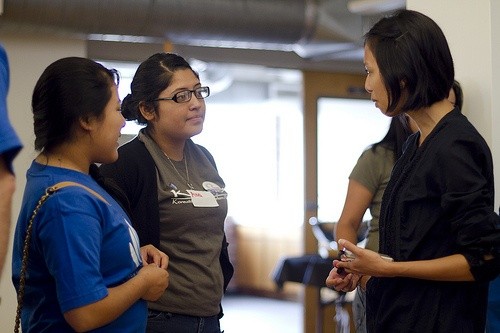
[344,248,393,262]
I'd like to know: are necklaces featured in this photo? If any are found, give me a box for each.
[163,149,197,189]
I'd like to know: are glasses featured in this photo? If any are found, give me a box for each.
[155,86,210,104]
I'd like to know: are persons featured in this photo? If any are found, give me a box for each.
[325,9,495,332]
[98,54,228,333]
[11,58,168,333]
[0,42,25,285]
